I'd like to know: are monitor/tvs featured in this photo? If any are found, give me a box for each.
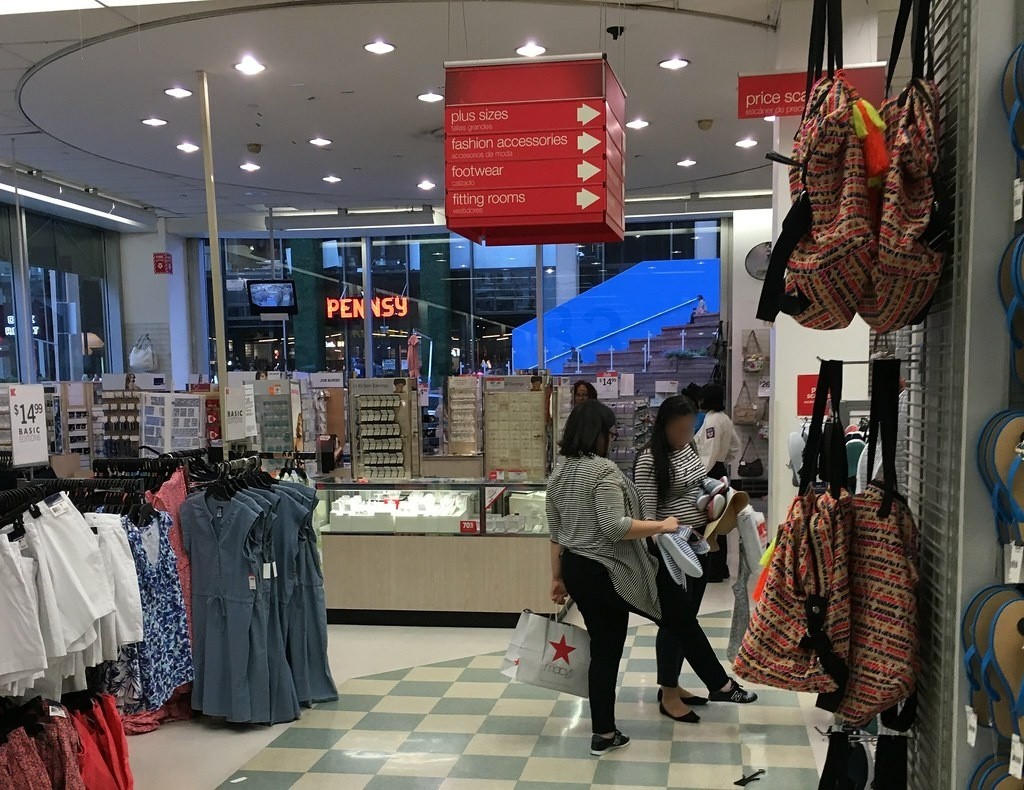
[246,280,298,316]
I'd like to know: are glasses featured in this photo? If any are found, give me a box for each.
[609,431,619,442]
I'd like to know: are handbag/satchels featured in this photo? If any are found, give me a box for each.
[831,358,921,729]
[500,602,591,698]
[733,381,756,425]
[857,0,943,331]
[732,356,853,695]
[709,364,726,399]
[707,321,727,360]
[757,399,769,438]
[758,379,770,397]
[738,436,763,477]
[744,331,763,372]
[737,504,767,570]
[130,334,159,371]
[755,0,891,330]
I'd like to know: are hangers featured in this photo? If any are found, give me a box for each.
[0,674,105,748]
[0,448,281,539]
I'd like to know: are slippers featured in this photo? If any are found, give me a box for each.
[962,42,1024,790]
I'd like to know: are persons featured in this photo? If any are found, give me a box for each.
[393,379,405,393]
[545,400,759,756]
[530,376,542,391]
[692,385,741,583]
[633,396,737,725]
[570,381,597,404]
[92,374,102,382]
[293,413,303,452]
[687,295,706,324]
[256,371,267,380]
[124,374,139,390]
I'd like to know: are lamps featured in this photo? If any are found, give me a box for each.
[0,165,156,229]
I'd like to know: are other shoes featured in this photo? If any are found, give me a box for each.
[785,432,807,486]
[656,525,710,592]
[657,686,708,723]
[696,476,728,522]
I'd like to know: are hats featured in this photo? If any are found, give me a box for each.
[700,485,750,553]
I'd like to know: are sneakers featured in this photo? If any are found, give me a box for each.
[709,678,757,703]
[590,729,631,756]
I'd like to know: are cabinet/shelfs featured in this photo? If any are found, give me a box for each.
[313,449,574,628]
[607,400,650,483]
